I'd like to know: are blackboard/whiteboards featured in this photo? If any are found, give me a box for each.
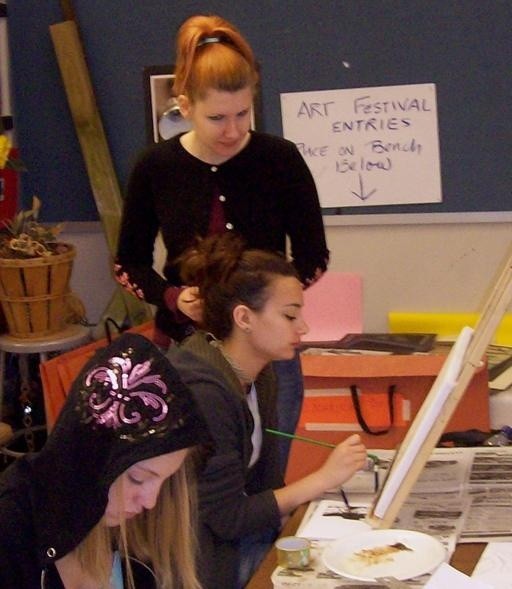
[1,1,511,235]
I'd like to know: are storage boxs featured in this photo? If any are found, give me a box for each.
[280,344,488,487]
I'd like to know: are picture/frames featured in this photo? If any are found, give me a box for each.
[145,60,265,147]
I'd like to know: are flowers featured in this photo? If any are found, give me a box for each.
[0,195,74,259]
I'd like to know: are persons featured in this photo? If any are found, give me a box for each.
[110,15,331,493]
[164,231,367,589]
[0,333,212,588]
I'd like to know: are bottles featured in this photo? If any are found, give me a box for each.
[485,425,511,447]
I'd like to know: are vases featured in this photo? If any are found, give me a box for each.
[0,243,77,339]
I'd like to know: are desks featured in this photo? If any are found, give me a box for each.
[0,323,91,459]
[242,427,512,589]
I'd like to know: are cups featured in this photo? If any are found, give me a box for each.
[274,536,311,570]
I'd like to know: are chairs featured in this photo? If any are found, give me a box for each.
[38,318,172,437]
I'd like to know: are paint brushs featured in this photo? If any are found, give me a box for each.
[374,464,379,494]
[340,485,352,514]
[265,428,393,463]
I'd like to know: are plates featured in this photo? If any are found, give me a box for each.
[320,529,446,583]
